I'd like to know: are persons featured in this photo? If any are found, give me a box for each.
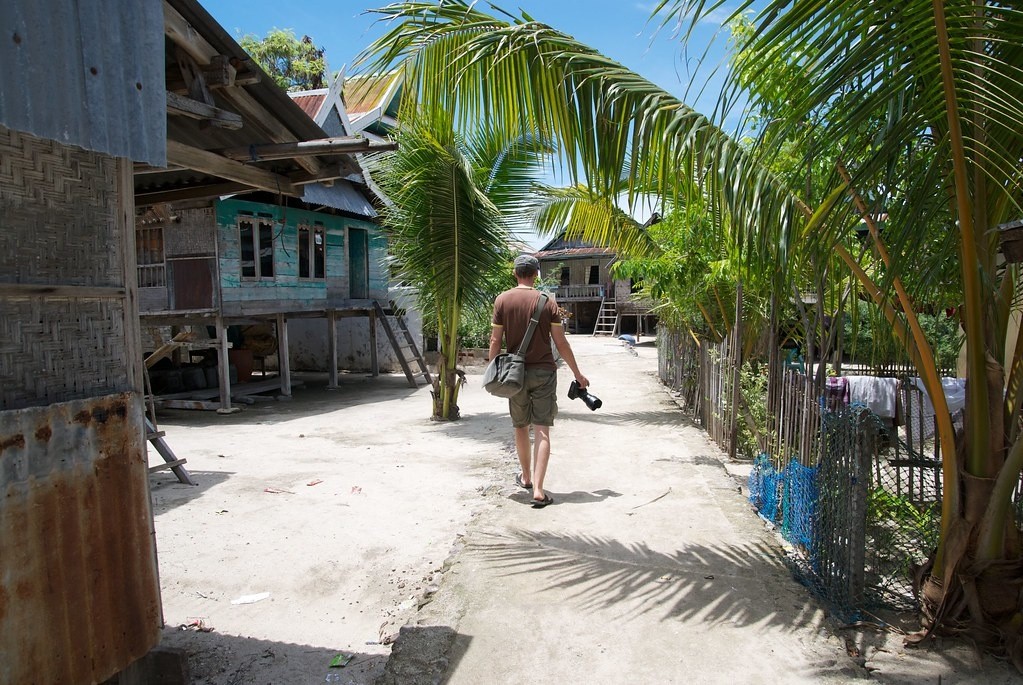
[488,255,590,505]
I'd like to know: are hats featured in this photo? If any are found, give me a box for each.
[514,255,540,269]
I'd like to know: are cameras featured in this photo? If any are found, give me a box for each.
[567,380,602,412]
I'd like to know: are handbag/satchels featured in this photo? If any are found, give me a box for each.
[481,353,524,398]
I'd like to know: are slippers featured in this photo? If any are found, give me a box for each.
[530,494,552,505]
[516,473,533,488]
